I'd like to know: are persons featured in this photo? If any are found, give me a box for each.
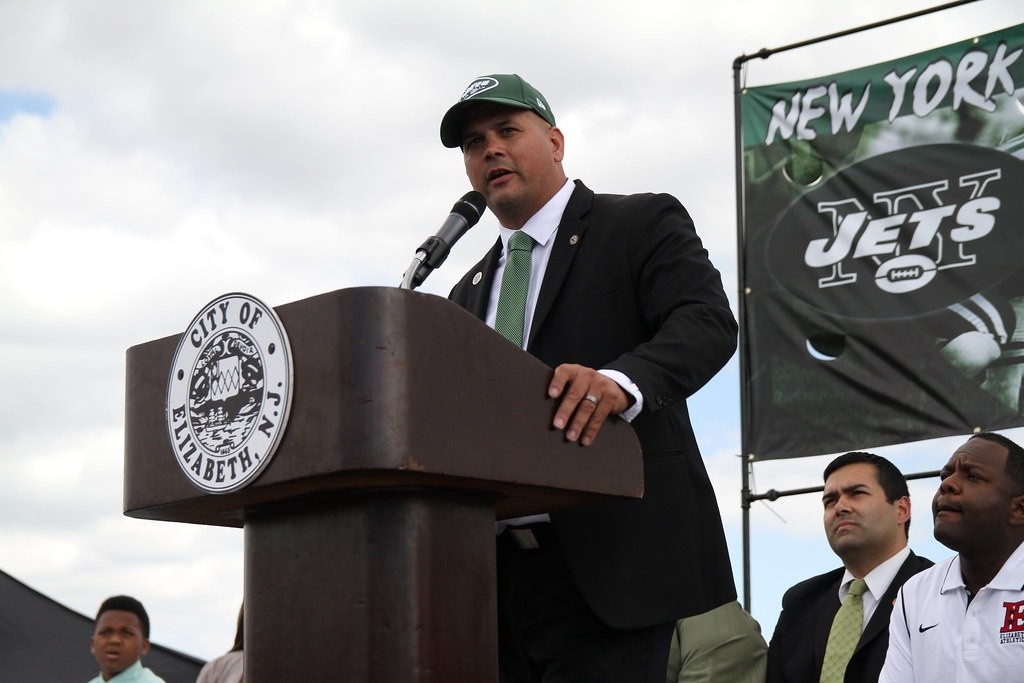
[668,432,1024,682]
[196,603,245,683]
[82,595,165,683]
[441,74,739,682]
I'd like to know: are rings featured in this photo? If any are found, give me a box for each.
[584,394,598,405]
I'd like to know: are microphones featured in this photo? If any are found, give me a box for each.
[399,190,487,290]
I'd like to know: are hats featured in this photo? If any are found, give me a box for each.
[440,73,556,148]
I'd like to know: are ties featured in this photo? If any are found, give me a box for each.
[819,579,869,683]
[495,231,538,349]
